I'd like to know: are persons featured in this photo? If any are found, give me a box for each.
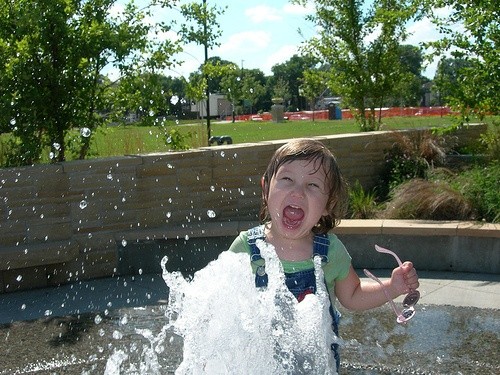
[228,139,420,375]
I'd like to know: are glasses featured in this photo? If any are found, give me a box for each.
[363,244,420,323]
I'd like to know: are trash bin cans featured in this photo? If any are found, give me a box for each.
[328,101,342,120]
